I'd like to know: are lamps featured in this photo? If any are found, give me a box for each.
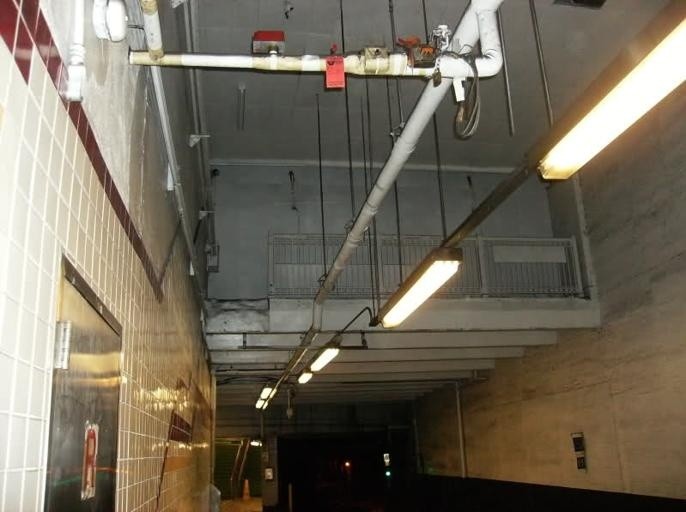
[379,247,463,336]
[310,344,340,373]
[255,386,273,410]
[298,370,314,387]
[526,20,686,180]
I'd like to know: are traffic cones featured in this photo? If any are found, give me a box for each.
[241,478,251,500]
